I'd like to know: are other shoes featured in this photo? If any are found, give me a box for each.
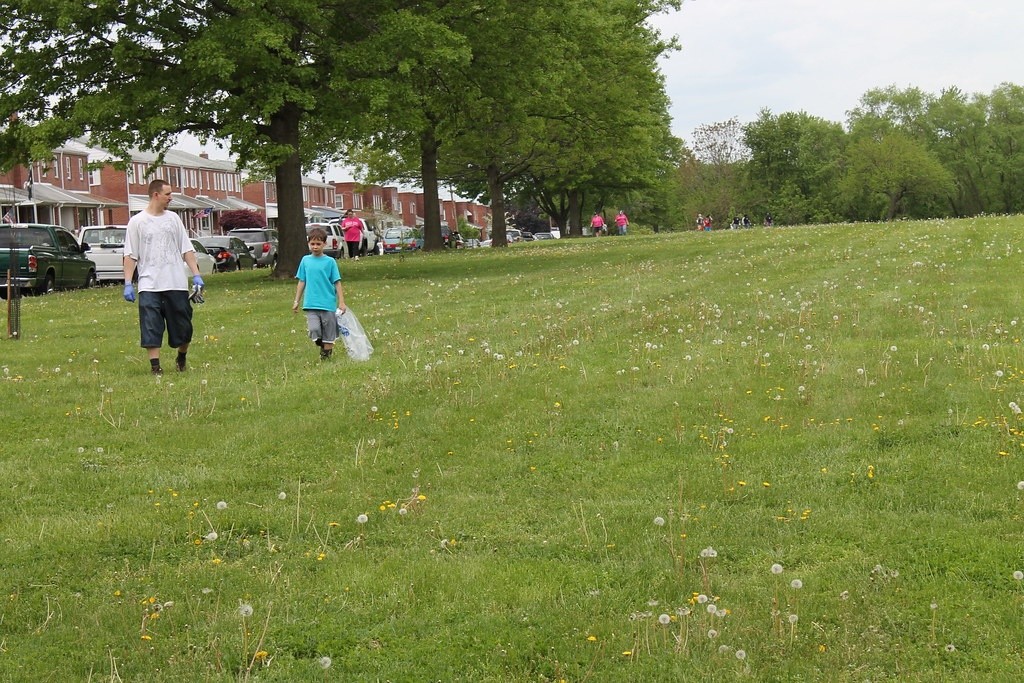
[151,365,163,375]
[320,349,333,361]
[355,257,359,261]
[175,358,186,372]
[351,257,354,262]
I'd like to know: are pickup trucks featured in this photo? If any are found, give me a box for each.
[0,223,97,296]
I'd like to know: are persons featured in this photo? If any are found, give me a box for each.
[589,212,605,237]
[696,212,773,232]
[448,231,464,250]
[123,179,205,376]
[342,209,367,261]
[292,229,346,361]
[614,210,630,236]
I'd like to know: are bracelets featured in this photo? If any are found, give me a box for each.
[295,301,299,303]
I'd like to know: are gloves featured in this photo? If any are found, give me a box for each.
[193,275,204,291]
[124,281,136,303]
[187,291,204,304]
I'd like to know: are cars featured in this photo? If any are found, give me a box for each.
[78,218,556,280]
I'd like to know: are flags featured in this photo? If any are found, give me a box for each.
[193,208,213,218]
[3,207,14,225]
[26,168,34,201]
